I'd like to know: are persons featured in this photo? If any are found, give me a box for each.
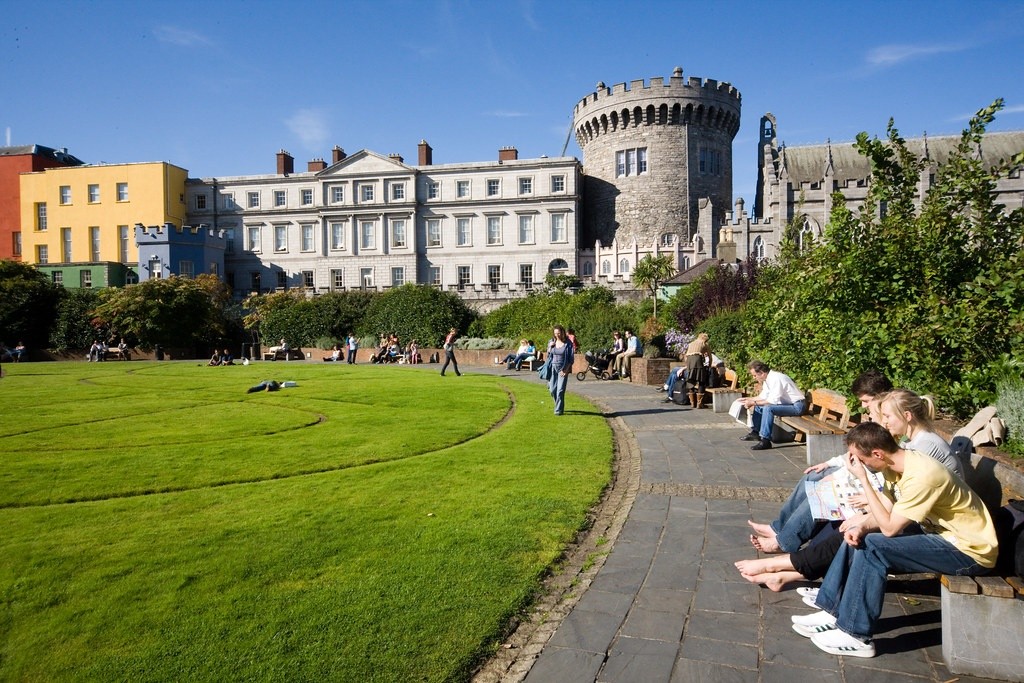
[610,331,644,380]
[88,340,108,362]
[346,333,360,365]
[566,328,576,353]
[655,333,726,409]
[12,342,24,363]
[739,360,808,450]
[498,339,536,371]
[247,380,281,394]
[118,338,128,361]
[369,333,418,365]
[323,346,343,361]
[732,372,998,658]
[440,328,465,377]
[271,339,289,361]
[547,325,574,415]
[209,349,234,366]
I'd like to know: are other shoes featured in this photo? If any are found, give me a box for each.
[623,372,628,378]
[612,371,621,375]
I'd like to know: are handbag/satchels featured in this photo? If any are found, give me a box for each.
[536,358,551,382]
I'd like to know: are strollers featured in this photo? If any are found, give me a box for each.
[576,342,615,380]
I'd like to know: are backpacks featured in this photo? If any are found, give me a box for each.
[673,379,688,405]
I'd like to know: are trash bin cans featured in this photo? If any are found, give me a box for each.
[241,342,251,360]
[252,343,261,360]
[154,344,164,359]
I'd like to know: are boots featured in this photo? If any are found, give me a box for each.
[688,392,696,410]
[697,392,706,410]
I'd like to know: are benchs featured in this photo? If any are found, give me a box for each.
[704,368,742,413]
[773,388,858,466]
[510,351,540,371]
[106,347,128,360]
[263,346,293,361]
[932,558,1024,683]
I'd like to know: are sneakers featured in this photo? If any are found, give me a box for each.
[811,635,877,660]
[791,613,843,638]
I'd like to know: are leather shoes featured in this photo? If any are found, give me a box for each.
[750,441,773,450]
[738,432,764,441]
